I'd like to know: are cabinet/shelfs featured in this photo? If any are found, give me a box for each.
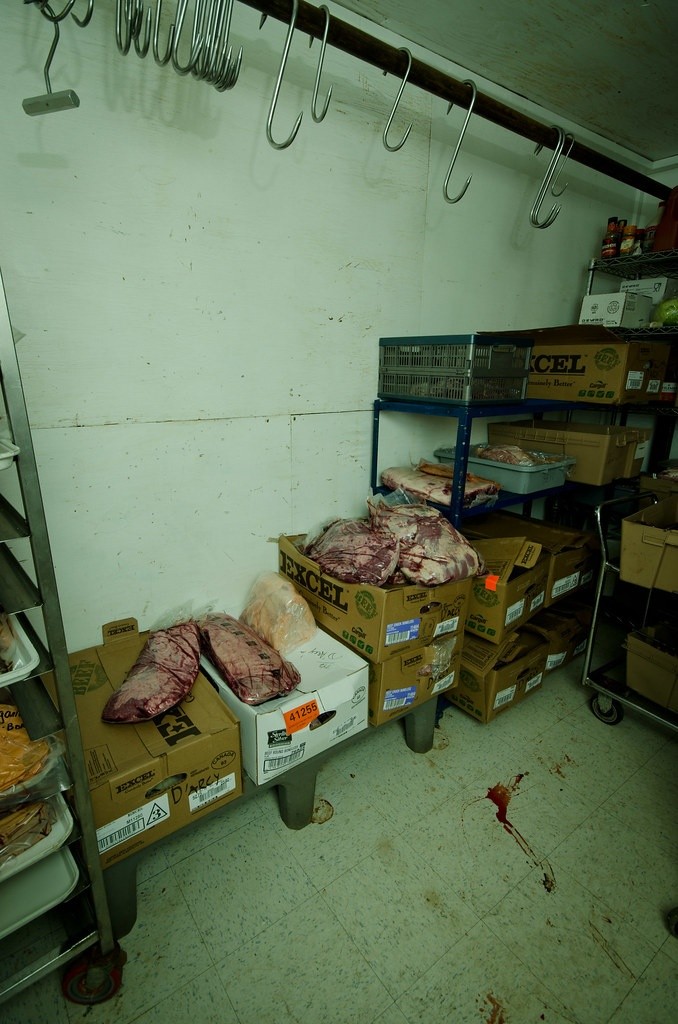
[0,270,130,1006]
[370,400,678,532]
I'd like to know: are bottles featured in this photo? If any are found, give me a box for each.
[601,185,678,266]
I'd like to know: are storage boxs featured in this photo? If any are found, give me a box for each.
[526,341,672,404]
[460,510,597,608]
[442,622,552,724]
[433,444,576,495]
[199,626,368,785]
[529,603,591,678]
[278,533,473,665]
[461,532,550,645]
[377,334,534,405]
[619,494,678,594]
[486,418,651,486]
[39,617,243,871]
[578,290,653,328]
[620,619,678,713]
[315,621,464,727]
[619,276,678,304]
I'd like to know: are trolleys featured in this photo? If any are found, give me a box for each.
[580,492,678,734]
